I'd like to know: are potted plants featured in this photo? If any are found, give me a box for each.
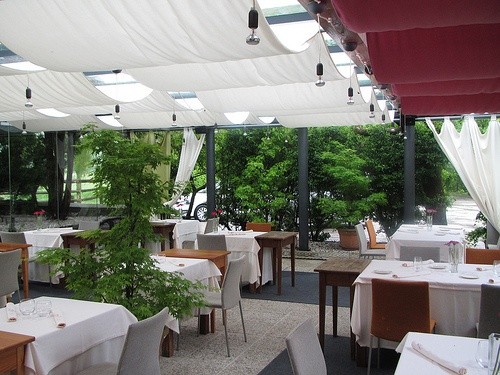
[318,139,386,250]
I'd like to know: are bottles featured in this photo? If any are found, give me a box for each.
[166,205,170,219]
[161,206,165,220]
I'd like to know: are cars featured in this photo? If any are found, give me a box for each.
[172,188,208,223]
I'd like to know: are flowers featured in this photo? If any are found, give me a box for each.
[211,208,222,219]
[425,208,437,220]
[34,210,45,218]
[445,240,460,249]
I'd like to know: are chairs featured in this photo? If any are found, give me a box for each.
[246,222,271,232]
[285,317,327,375]
[183,219,214,250]
[365,219,387,249]
[176,255,247,357]
[399,245,441,263]
[355,224,386,259]
[76,307,170,375]
[366,278,437,375]
[0,248,23,303]
[197,234,227,252]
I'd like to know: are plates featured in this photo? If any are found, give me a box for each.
[375,270,391,274]
[430,265,446,269]
[459,275,478,279]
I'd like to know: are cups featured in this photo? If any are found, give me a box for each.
[19,299,35,316]
[150,252,166,264]
[494,261,500,277]
[477,340,489,368]
[36,300,52,317]
[414,257,422,272]
[49,223,59,232]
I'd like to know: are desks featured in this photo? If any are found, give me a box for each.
[0,223,500,375]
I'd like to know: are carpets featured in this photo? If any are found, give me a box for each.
[6,266,77,303]
[256,333,401,375]
[240,270,354,307]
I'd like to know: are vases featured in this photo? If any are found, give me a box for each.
[36,216,43,232]
[426,216,434,229]
[449,245,459,273]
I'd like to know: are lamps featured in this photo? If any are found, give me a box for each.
[25,0,388,125]
